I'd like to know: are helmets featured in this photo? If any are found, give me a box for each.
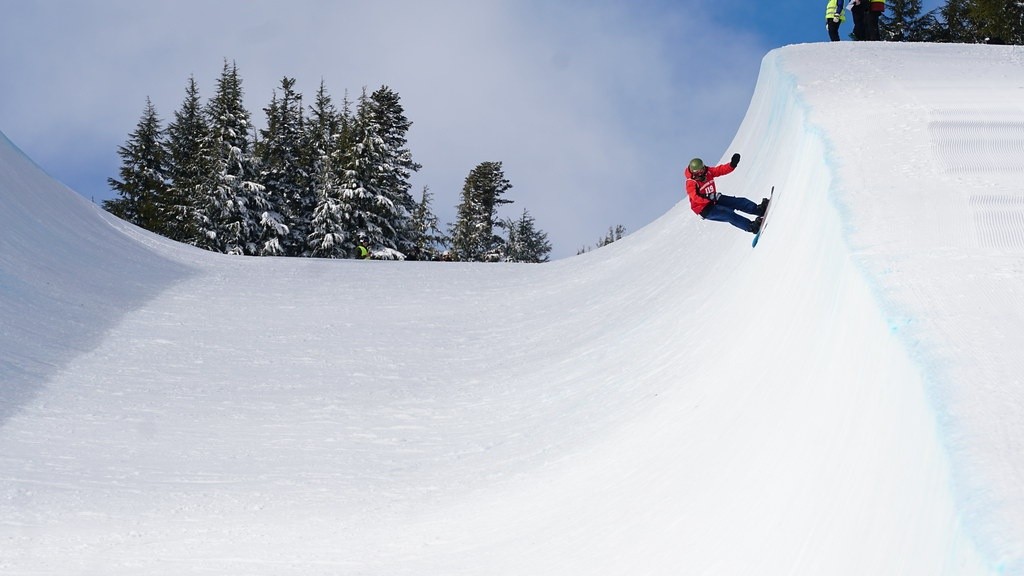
[690,158,704,174]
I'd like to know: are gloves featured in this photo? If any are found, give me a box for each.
[825,24,829,30]
[730,154,741,168]
[833,13,840,22]
[708,200,715,207]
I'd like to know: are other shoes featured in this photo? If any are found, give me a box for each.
[755,204,765,217]
[751,217,762,234]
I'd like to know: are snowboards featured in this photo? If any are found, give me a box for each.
[751,185,775,248]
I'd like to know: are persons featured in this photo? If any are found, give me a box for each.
[825,0,885,42]
[354,240,372,260]
[685,152,771,235]
